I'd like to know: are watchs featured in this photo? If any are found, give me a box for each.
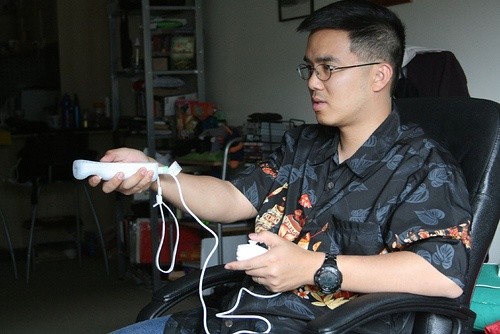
[313,251,344,295]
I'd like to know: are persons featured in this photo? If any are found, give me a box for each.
[89,1,473,334]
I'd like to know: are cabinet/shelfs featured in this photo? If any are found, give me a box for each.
[110,1,206,150]
[167,136,249,273]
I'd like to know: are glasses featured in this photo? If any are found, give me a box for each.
[296,62,381,81]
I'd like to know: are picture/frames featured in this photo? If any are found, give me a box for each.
[276,0,314,21]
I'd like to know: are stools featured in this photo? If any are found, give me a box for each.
[19,135,110,283]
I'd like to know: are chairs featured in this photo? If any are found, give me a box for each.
[135,98,500,334]
